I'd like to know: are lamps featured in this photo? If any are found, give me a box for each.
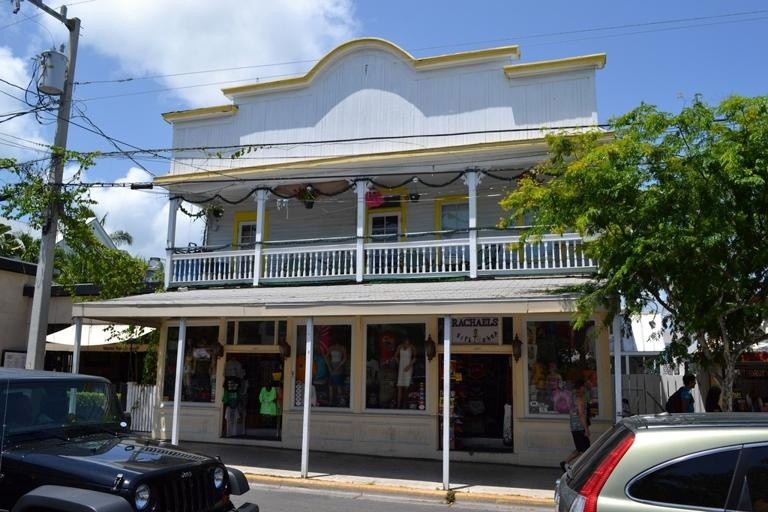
[512,333,523,362]
[426,334,436,362]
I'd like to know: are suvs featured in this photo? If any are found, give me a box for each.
[0,366,260,512]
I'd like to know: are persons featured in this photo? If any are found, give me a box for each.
[545,361,564,410]
[394,339,418,410]
[705,385,722,412]
[324,340,346,405]
[559,384,592,472]
[679,373,697,412]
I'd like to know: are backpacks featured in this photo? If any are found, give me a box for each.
[666,387,683,413]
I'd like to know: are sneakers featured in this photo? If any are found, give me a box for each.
[560,461,570,472]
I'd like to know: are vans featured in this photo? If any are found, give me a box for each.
[553,411,767,511]
